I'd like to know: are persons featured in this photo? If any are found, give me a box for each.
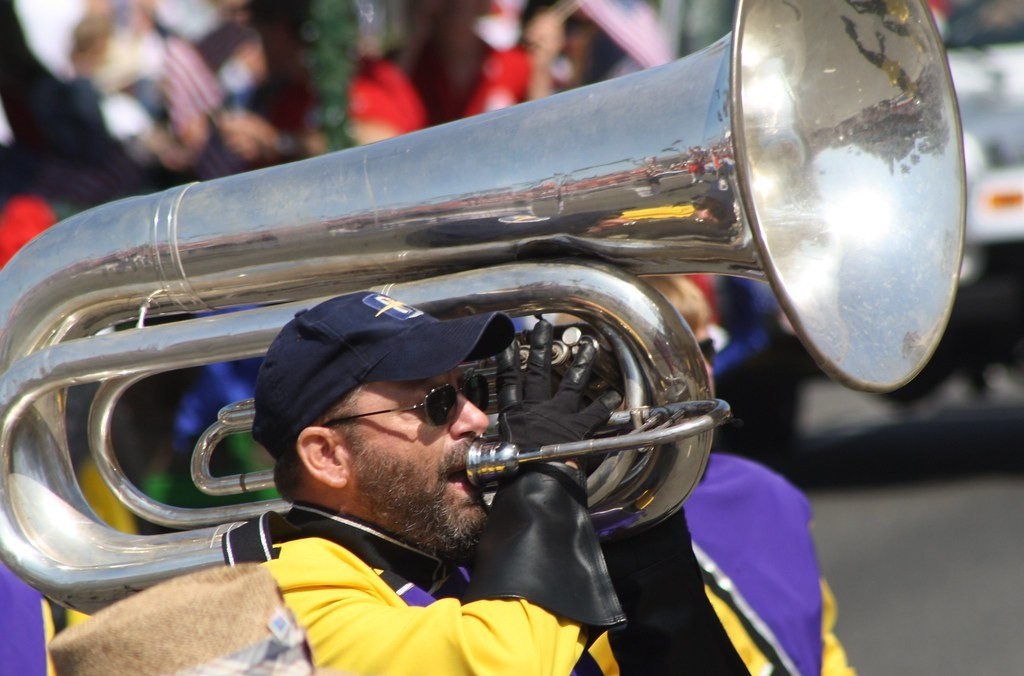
[404,127,739,247]
[842,0,929,105]
[0,291,856,676]
[0,1,679,276]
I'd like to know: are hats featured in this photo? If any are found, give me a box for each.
[250,291,515,460]
[47,559,341,676]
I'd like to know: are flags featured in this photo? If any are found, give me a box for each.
[154,24,221,128]
[579,0,676,70]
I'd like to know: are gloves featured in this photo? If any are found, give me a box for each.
[462,319,627,627]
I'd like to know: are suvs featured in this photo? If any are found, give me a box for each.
[713,43,1024,455]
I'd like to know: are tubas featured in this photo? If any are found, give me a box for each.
[1,0,968,621]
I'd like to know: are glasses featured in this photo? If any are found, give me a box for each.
[322,375,489,427]
[696,337,714,365]
